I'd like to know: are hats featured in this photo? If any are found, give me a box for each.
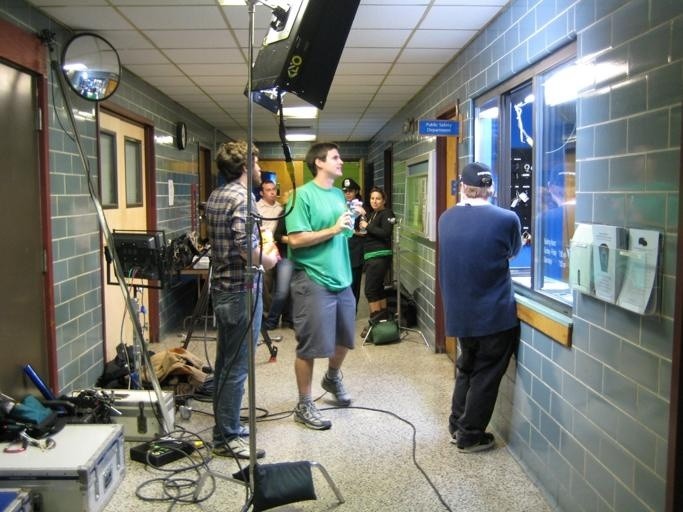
[457,161,493,188]
[341,177,359,192]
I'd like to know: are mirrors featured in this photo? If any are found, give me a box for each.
[60,33,121,102]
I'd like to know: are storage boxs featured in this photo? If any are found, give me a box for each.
[0,388,176,512]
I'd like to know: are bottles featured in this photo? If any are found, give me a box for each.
[347,206,358,240]
[359,213,368,235]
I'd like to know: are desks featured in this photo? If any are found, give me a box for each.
[180,270,218,349]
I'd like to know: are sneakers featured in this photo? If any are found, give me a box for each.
[320,374,351,407]
[211,436,264,459]
[237,424,249,435]
[448,432,497,454]
[294,401,331,430]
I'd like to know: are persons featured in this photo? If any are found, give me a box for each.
[598,244,609,272]
[342,179,364,317]
[358,184,397,338]
[266,189,300,331]
[439,161,521,452]
[256,181,282,315]
[206,140,265,460]
[281,143,352,431]
[539,166,576,282]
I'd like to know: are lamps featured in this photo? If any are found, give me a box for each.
[193,0,361,512]
